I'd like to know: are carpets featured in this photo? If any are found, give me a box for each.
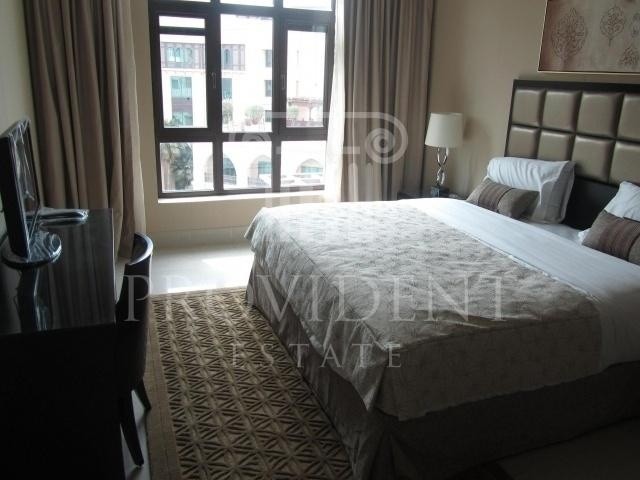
[142,285,511,479]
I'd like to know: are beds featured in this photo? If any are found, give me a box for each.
[243,73,639,479]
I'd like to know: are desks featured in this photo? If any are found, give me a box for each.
[1,206,128,480]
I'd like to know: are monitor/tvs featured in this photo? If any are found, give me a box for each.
[0,118,63,270]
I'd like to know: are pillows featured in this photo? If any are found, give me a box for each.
[577,180,639,246]
[466,176,540,219]
[486,155,577,226]
[582,210,639,263]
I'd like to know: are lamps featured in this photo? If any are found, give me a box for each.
[419,110,467,198]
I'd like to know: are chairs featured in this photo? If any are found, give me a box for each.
[113,230,153,469]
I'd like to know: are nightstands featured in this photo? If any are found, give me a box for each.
[396,189,465,200]
